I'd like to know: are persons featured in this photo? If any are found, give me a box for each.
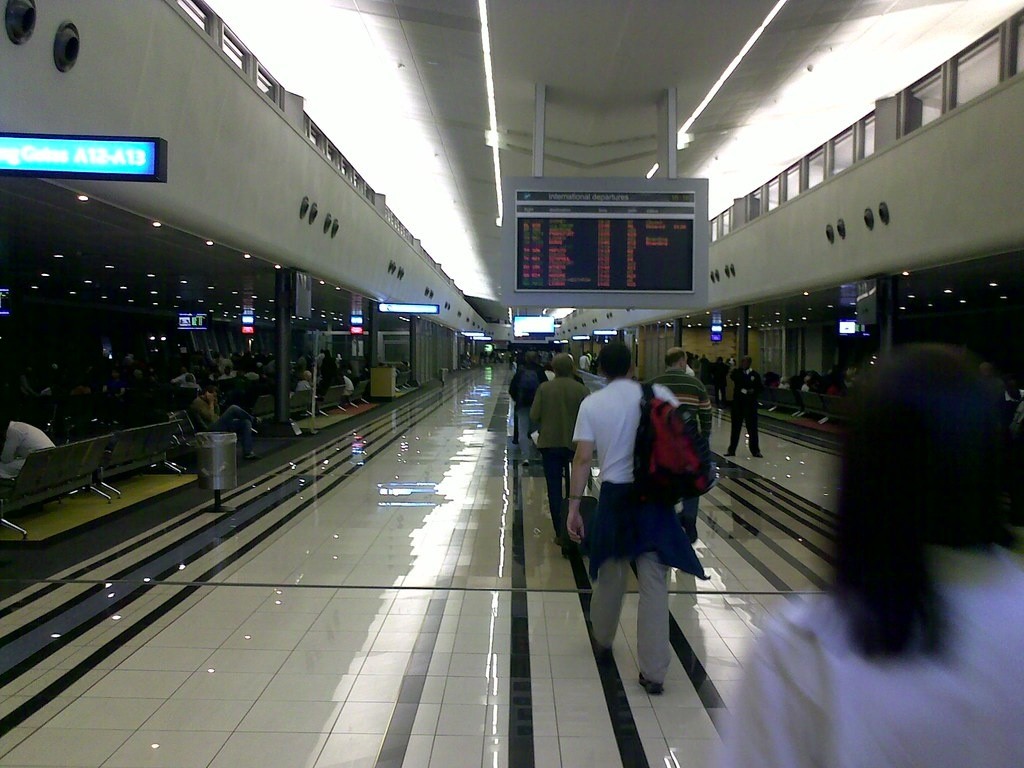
[454,339,1024,768]
[1,347,423,482]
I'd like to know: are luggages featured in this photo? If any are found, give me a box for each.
[562,495,599,558]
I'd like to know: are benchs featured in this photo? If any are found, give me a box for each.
[760,386,845,423]
[251,369,412,417]
[0,408,205,539]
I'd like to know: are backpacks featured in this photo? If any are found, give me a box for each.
[633,381,718,502]
[519,365,542,403]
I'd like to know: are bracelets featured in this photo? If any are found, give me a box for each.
[568,494,582,500]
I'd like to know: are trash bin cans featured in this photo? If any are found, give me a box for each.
[370,367,396,401]
[196,432,238,512]
[439,368,448,387]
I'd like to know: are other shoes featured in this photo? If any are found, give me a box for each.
[723,453,735,456]
[255,416,265,425]
[597,647,611,668]
[243,452,256,459]
[512,441,518,444]
[639,672,662,694]
[753,453,763,458]
[523,459,529,466]
